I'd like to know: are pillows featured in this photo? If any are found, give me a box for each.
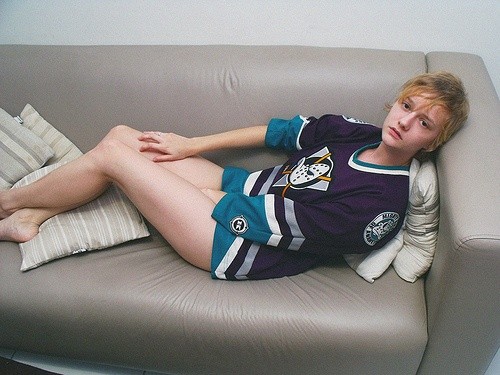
[16,102,84,167]
[0,108,56,190]
[342,158,421,283]
[10,159,150,272]
[391,156,440,283]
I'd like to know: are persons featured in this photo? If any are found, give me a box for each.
[0,69,468,283]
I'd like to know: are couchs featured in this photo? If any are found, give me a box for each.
[0,43,500,375]
